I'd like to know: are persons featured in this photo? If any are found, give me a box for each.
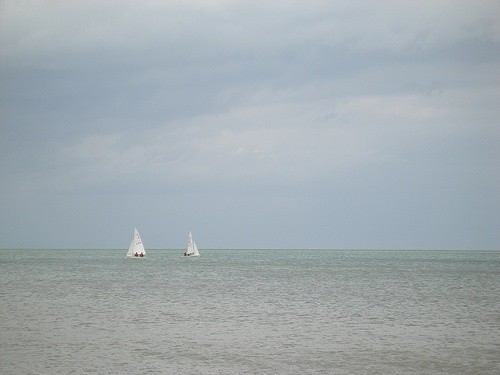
[135,252,138,256]
[140,252,143,256]
[184,252,186,256]
[188,253,189,256]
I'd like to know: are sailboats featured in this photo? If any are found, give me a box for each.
[124,227,146,259]
[180,232,200,257]
